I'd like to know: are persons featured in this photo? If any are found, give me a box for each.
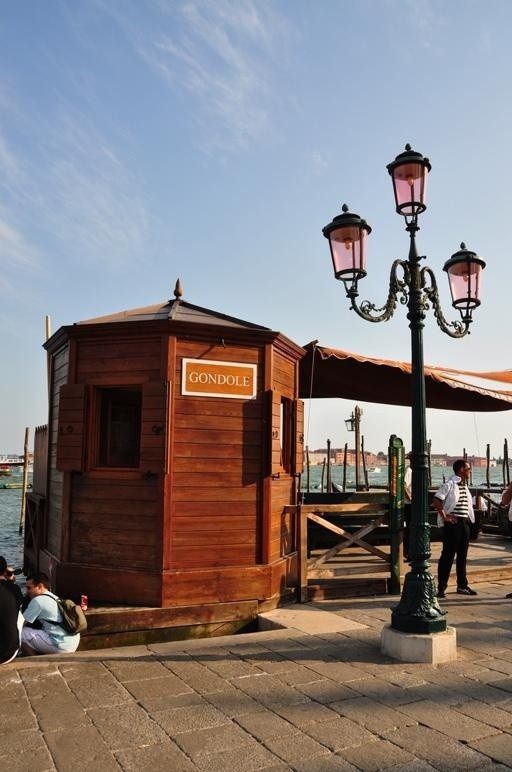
[403,452,412,562]
[499,480,511,600]
[0,556,26,665]
[433,460,476,597]
[21,572,81,656]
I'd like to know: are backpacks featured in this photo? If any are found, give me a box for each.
[45,594,90,637]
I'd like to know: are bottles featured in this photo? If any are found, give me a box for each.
[79,594,90,611]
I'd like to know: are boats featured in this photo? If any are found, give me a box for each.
[0,454,34,478]
[366,466,382,475]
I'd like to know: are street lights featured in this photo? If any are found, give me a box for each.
[321,140,487,664]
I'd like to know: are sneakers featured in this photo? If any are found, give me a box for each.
[436,586,449,599]
[455,582,477,597]
